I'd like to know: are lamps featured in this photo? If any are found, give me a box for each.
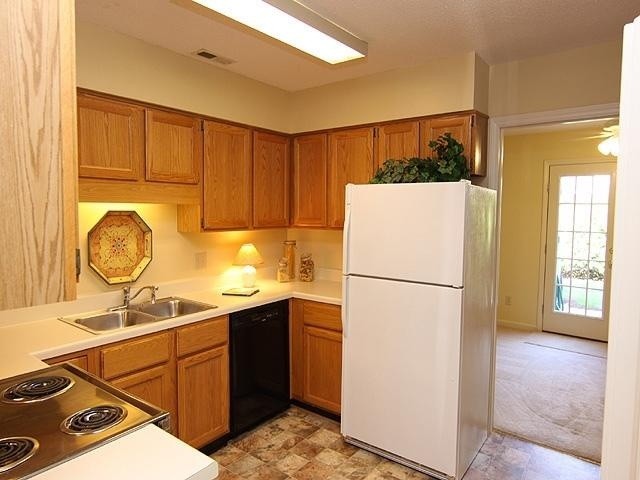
[597,134,619,156]
[232,244,263,288]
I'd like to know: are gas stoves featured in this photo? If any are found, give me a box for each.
[0,363,168,480]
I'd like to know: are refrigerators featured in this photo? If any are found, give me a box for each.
[339,180,499,479]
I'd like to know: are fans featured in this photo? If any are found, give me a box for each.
[562,121,619,145]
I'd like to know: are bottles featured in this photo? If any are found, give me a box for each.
[277,240,316,283]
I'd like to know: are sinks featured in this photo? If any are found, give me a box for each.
[58,305,155,335]
[140,297,218,322]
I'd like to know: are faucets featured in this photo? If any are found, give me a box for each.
[124,286,158,304]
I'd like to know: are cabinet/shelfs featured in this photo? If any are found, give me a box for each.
[179,120,291,235]
[300,302,343,414]
[377,112,491,176]
[100,331,170,441]
[290,124,377,231]
[77,89,200,187]
[177,315,231,452]
